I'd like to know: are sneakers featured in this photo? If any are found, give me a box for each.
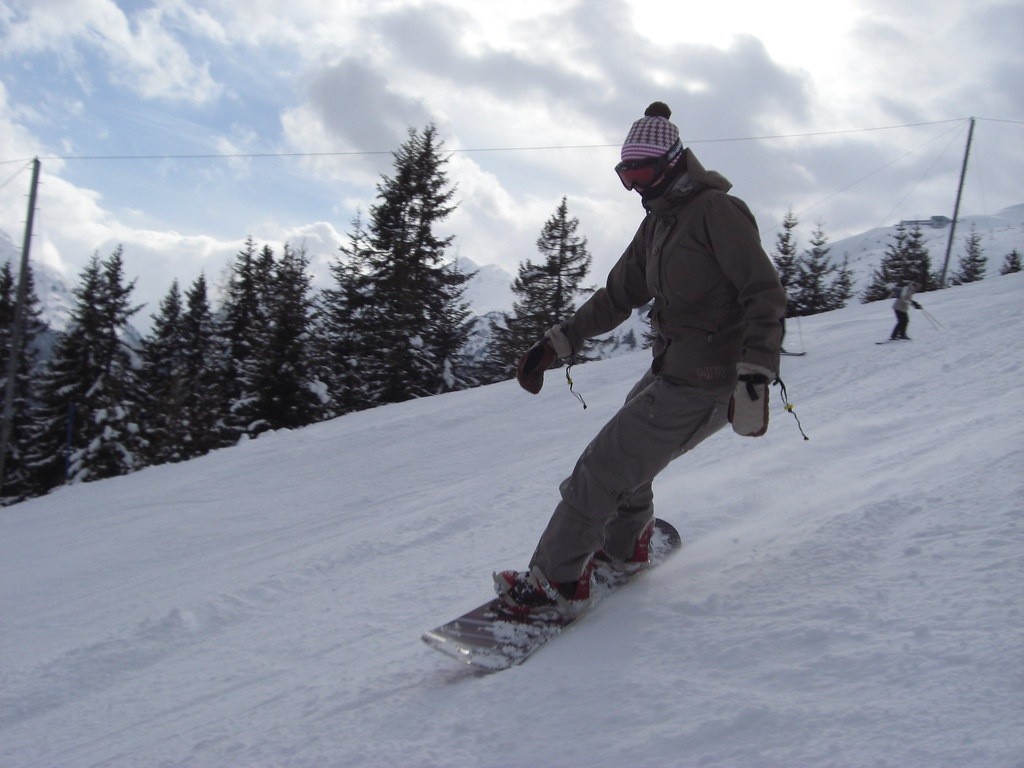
[588,518,655,571]
[493,566,594,618]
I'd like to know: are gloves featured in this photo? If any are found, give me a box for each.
[914,303,922,311]
[517,324,574,394]
[726,363,777,438]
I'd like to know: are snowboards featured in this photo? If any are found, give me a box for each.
[422,518,684,670]
[781,350,807,357]
[875,337,896,346]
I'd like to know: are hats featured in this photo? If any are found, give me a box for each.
[620,101,683,168]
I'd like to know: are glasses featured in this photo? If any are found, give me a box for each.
[615,157,667,191]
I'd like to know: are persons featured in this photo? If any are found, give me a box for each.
[890,276,923,340]
[493,99,786,620]
